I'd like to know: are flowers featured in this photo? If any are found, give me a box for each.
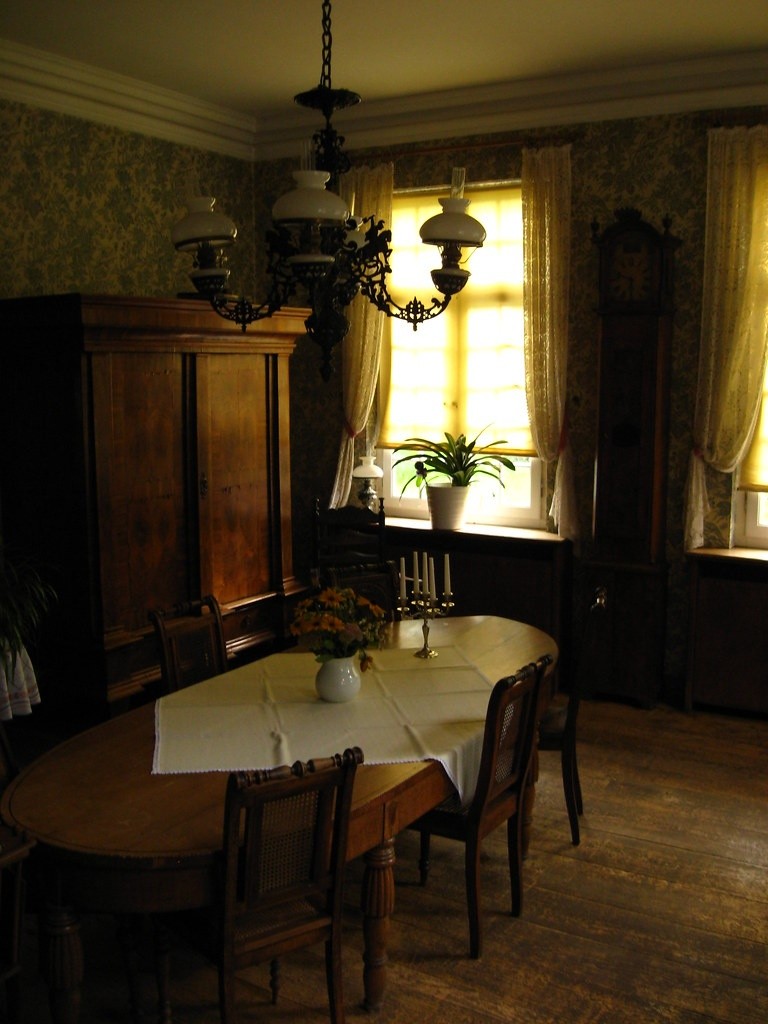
[289,570,386,673]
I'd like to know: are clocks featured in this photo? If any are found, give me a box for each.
[589,205,684,318]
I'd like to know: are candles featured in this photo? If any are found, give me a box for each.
[429,557,436,600]
[400,557,406,599]
[444,554,451,593]
[413,551,419,592]
[422,552,428,593]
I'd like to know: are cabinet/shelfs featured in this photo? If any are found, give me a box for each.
[0,291,313,738]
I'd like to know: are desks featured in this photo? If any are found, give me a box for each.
[0,613,561,1024]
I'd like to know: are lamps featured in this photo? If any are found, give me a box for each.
[170,0,487,384]
[352,454,384,511]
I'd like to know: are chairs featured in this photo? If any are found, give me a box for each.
[148,747,364,1024]
[312,494,387,585]
[146,595,230,694]
[324,559,402,622]
[405,655,554,961]
[534,583,608,846]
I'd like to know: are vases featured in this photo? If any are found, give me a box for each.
[314,659,362,704]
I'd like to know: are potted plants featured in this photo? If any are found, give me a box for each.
[390,423,516,531]
